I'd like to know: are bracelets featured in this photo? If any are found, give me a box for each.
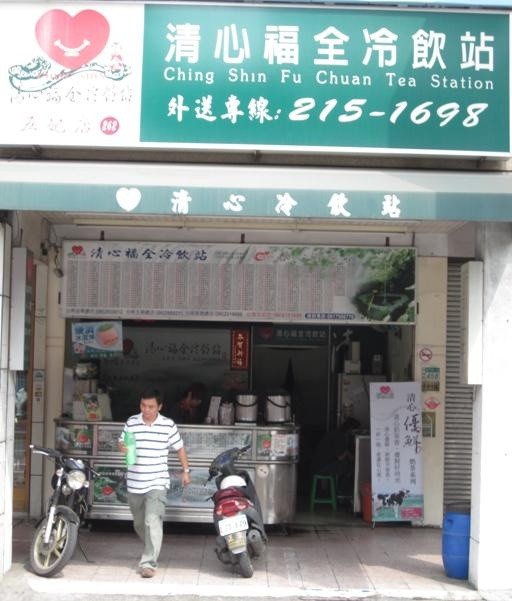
[182,469,191,473]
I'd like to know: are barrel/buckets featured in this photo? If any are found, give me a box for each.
[235,387,291,423]
[361,481,371,523]
[442,502,470,579]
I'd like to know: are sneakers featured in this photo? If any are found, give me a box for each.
[142,567,154,577]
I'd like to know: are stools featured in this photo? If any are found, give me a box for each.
[309,474,338,514]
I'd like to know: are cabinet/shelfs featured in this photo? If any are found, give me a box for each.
[342,428,371,518]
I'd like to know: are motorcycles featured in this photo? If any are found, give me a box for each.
[26,445,123,576]
[204,445,269,579]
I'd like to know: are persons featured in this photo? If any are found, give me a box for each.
[168,382,208,424]
[117,388,191,578]
[312,417,360,499]
